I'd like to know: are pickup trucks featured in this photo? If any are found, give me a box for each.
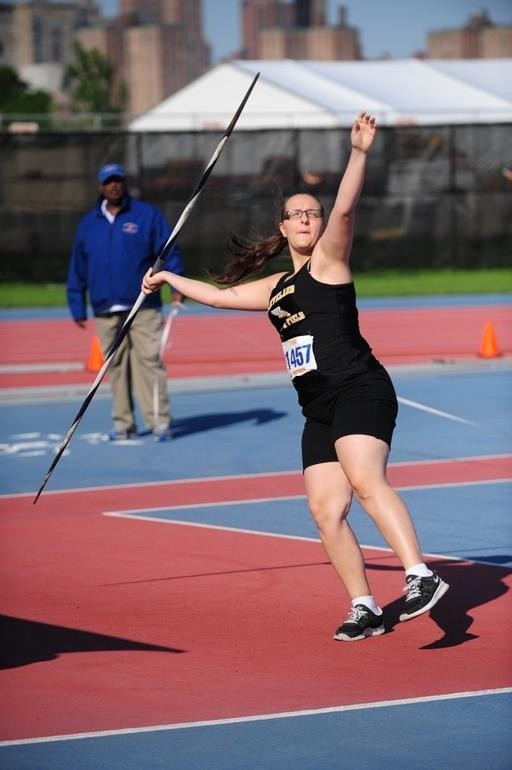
[136,157,233,201]
[236,155,322,197]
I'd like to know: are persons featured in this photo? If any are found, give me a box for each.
[66,163,188,445]
[141,109,450,641]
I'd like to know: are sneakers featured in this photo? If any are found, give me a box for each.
[333,603,386,642]
[399,572,449,621]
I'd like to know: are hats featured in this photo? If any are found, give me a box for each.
[99,163,125,183]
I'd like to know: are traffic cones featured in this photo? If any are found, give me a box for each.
[85,334,105,373]
[476,320,504,357]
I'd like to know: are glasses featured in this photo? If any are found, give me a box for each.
[281,210,323,222]
